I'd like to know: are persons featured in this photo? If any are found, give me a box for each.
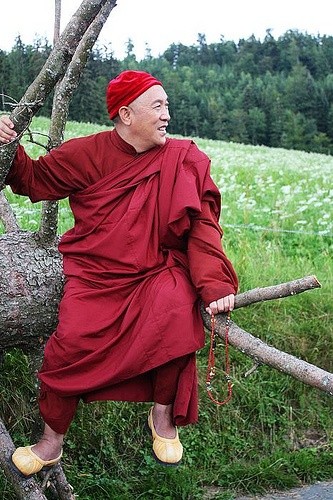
[0,71,239,477]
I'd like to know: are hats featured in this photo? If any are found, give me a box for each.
[106,70,162,120]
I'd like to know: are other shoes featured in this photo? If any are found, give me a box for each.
[10,443,63,479]
[147,406,183,466]
